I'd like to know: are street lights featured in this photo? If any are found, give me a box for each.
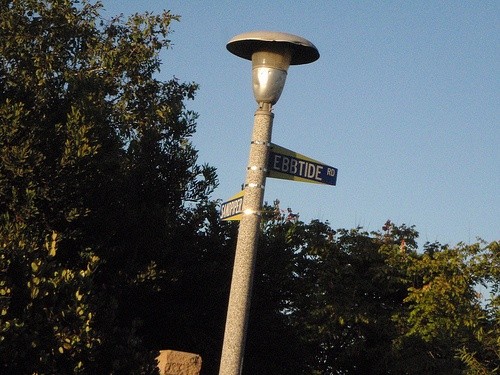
[211,30,322,375]
